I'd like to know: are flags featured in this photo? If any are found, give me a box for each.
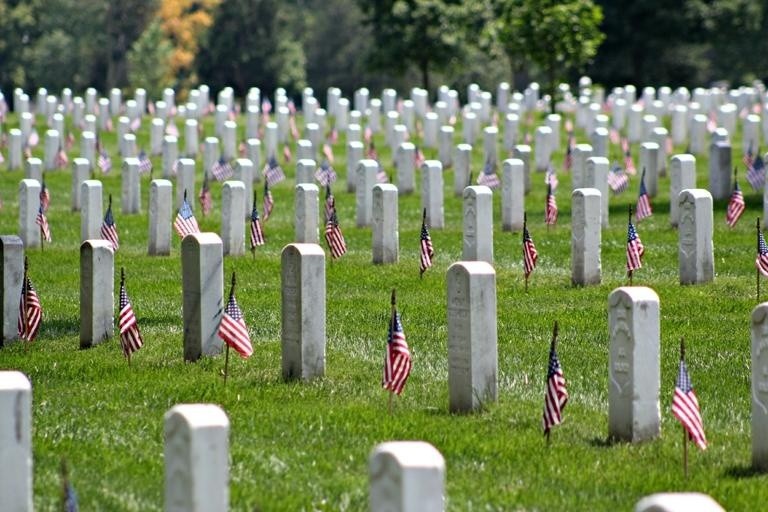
[752,231,768,276]
[99,205,119,253]
[625,221,647,279]
[216,293,256,360]
[5,94,767,233]
[249,207,265,250]
[118,286,144,357]
[380,308,413,396]
[175,200,200,242]
[324,209,346,259]
[418,223,435,276]
[538,347,572,432]
[519,227,539,277]
[16,266,41,342]
[670,356,708,453]
[36,185,53,242]
